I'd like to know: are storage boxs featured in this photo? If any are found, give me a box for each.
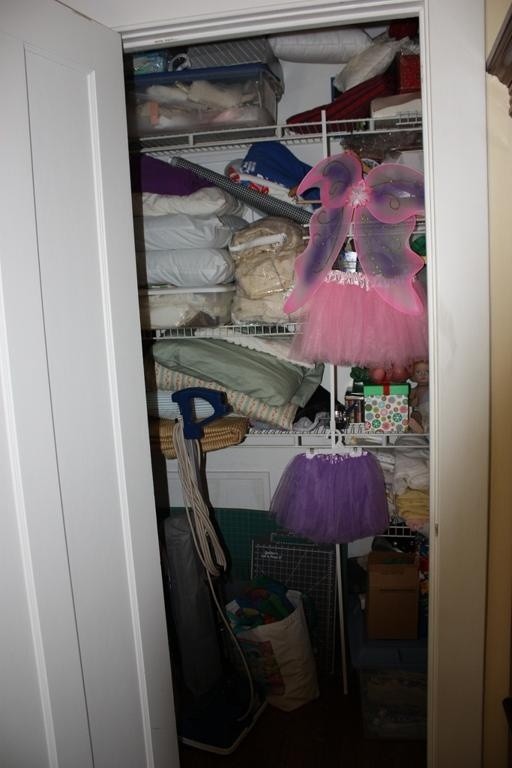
[367,564,420,641]
[135,63,286,140]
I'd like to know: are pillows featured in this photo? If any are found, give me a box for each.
[270,27,373,64]
[142,187,247,218]
[155,361,300,431]
[332,37,409,92]
[144,216,250,248]
[145,247,237,287]
[151,337,305,405]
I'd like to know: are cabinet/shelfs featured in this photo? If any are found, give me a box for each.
[137,107,432,526]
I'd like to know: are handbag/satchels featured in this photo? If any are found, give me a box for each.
[230,587,323,714]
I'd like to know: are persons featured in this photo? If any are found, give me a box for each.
[407,360,429,435]
